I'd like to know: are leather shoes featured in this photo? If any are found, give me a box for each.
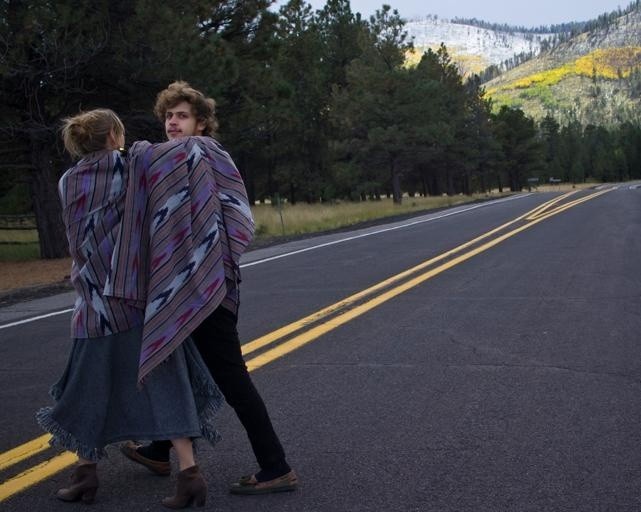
[119,440,171,474]
[230,470,297,495]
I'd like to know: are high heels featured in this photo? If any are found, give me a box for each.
[161,465,207,507]
[57,463,98,503]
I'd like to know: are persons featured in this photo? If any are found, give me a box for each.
[118,80,300,494]
[36,110,257,508]
